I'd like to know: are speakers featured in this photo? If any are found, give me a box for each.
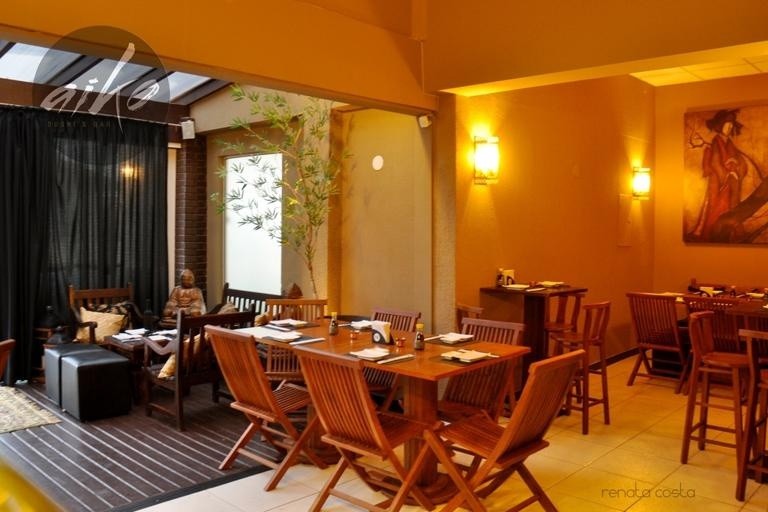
[180,117,196,140]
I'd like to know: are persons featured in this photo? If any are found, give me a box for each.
[162,268,206,320]
[688,108,749,242]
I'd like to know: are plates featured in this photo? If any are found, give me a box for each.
[537,280,564,287]
[502,283,529,290]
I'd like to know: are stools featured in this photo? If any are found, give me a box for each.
[42,341,132,423]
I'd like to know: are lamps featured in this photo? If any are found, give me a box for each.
[631,167,652,202]
[473,134,500,186]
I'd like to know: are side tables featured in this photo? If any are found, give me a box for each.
[32,327,64,383]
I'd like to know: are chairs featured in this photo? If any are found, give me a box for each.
[67,284,286,434]
[624,281,768,501]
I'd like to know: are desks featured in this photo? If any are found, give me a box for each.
[480,285,587,401]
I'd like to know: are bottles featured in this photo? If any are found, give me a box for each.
[729,284,736,297]
[413,323,425,350]
[328,311,338,337]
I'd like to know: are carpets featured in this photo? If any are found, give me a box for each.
[0,385,63,436]
